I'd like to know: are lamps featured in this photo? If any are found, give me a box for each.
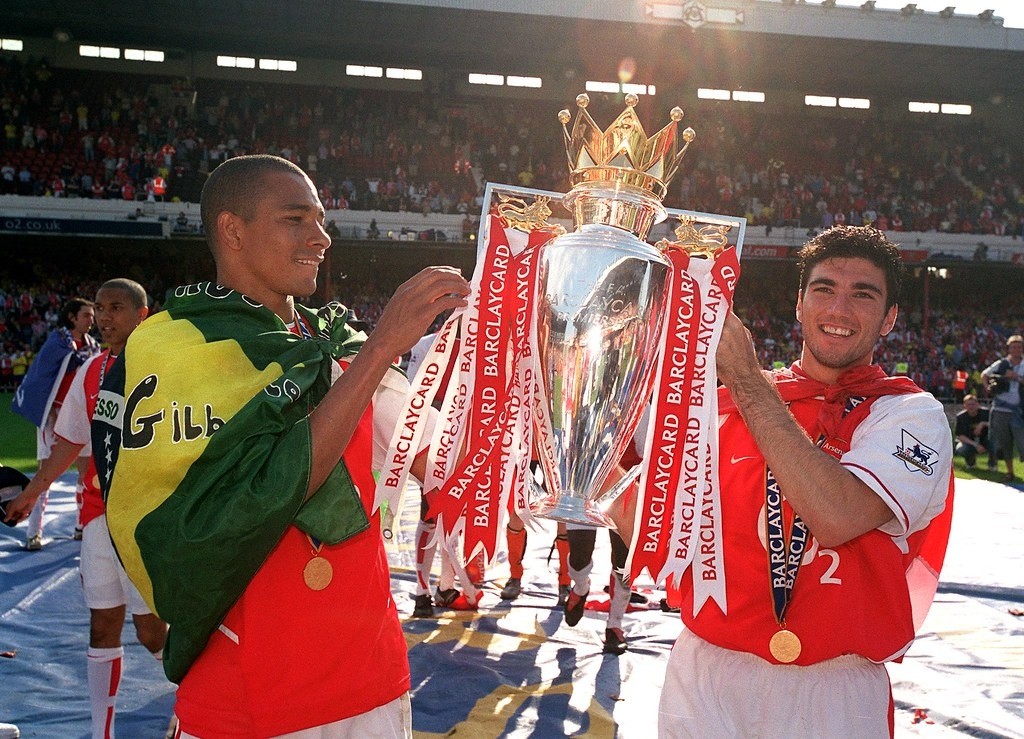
[821,0,837,7]
[900,3,917,16]
[860,0,876,11]
[977,10,994,19]
[939,6,955,18]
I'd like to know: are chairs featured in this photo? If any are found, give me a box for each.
[0,119,141,198]
[333,148,386,182]
[256,126,310,154]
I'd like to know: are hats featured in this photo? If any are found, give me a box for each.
[0,466,33,528]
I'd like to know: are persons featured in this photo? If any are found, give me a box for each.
[0,68,1024,739]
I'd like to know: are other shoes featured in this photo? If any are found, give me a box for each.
[74,526,83,540]
[26,536,44,551]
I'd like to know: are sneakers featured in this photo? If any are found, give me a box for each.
[434,586,459,607]
[564,577,592,626]
[500,577,522,599]
[604,624,629,654]
[555,585,572,606]
[409,591,434,618]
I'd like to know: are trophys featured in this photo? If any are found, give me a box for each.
[524,89,697,528]
